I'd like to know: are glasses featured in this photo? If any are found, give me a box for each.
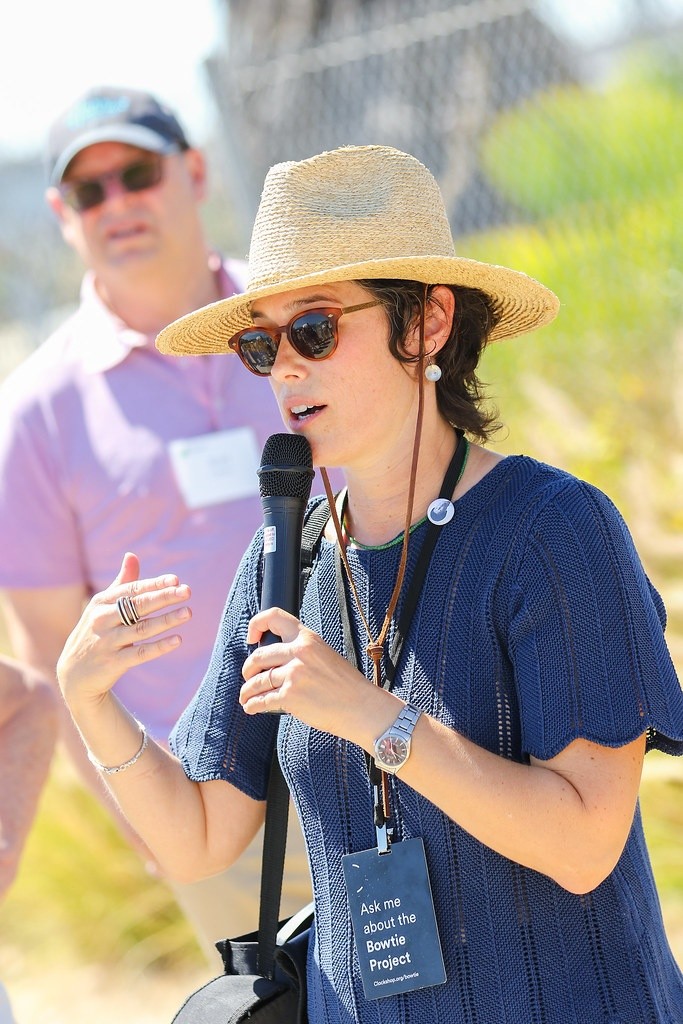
[57,155,164,213]
[227,300,387,377]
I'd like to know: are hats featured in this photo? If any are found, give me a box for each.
[43,85,190,190]
[153,143,562,358]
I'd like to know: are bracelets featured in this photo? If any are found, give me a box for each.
[87,723,148,775]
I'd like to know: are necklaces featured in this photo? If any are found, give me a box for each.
[342,437,470,550]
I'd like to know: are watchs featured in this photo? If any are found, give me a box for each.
[372,702,423,775]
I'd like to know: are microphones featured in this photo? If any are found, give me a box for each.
[257,433,315,649]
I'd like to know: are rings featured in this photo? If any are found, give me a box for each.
[268,667,275,689]
[117,595,140,626]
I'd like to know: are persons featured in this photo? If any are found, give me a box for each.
[0,85,345,1024]
[56,144,683,1024]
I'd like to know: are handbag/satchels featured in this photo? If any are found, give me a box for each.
[168,902,320,1024]
